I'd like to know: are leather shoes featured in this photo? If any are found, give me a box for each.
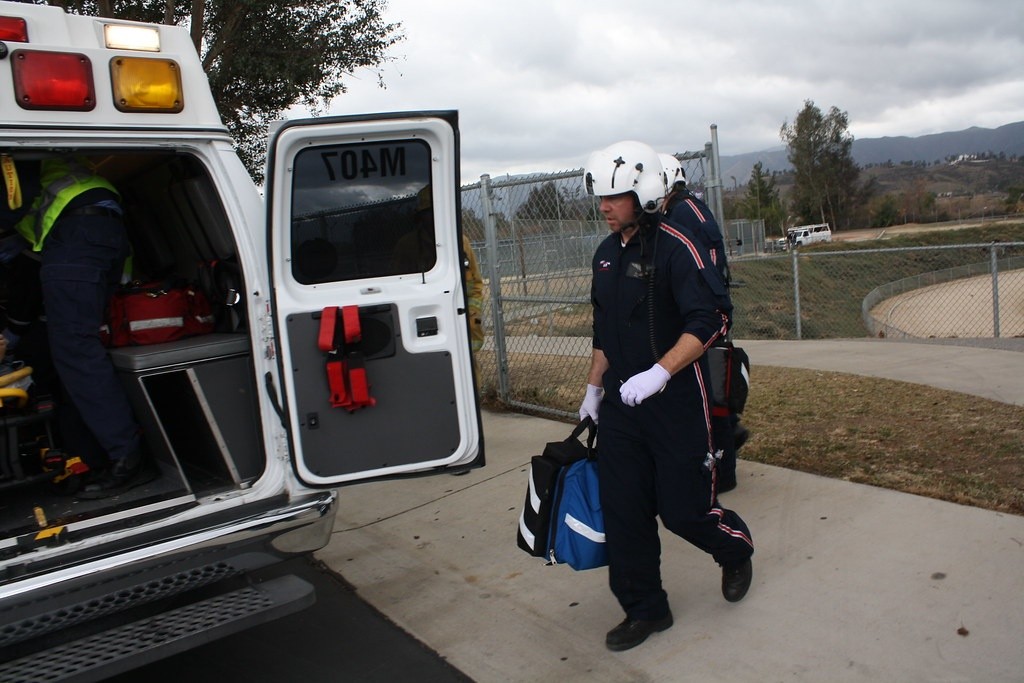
[606,604,673,651]
[84,440,162,500]
[719,552,752,602]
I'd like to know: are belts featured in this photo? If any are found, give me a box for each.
[65,206,121,221]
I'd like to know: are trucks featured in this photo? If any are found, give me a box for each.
[779,222,832,251]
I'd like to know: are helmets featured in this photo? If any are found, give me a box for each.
[658,153,686,196]
[583,141,665,214]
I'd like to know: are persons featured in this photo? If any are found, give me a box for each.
[450,235,484,476]
[579,140,755,651]
[388,186,435,274]
[0,150,157,500]
[658,153,750,493]
[735,237,743,256]
[789,231,797,246]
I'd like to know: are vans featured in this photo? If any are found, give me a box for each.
[1,2,488,683]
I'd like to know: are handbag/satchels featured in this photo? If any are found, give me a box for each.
[517,415,599,557]
[98,261,215,348]
[707,342,750,414]
[544,418,610,571]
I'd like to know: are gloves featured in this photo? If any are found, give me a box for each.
[619,363,672,407]
[579,383,604,425]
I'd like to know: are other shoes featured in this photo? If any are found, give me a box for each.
[716,468,737,494]
[733,423,749,451]
